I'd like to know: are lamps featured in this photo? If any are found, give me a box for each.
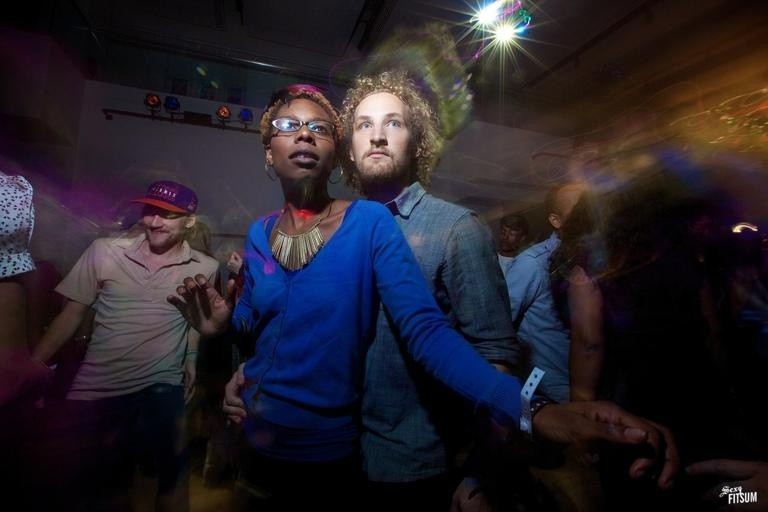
[144,92,254,133]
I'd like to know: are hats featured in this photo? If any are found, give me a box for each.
[132,180,197,214]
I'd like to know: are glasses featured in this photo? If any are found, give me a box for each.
[269,117,337,133]
